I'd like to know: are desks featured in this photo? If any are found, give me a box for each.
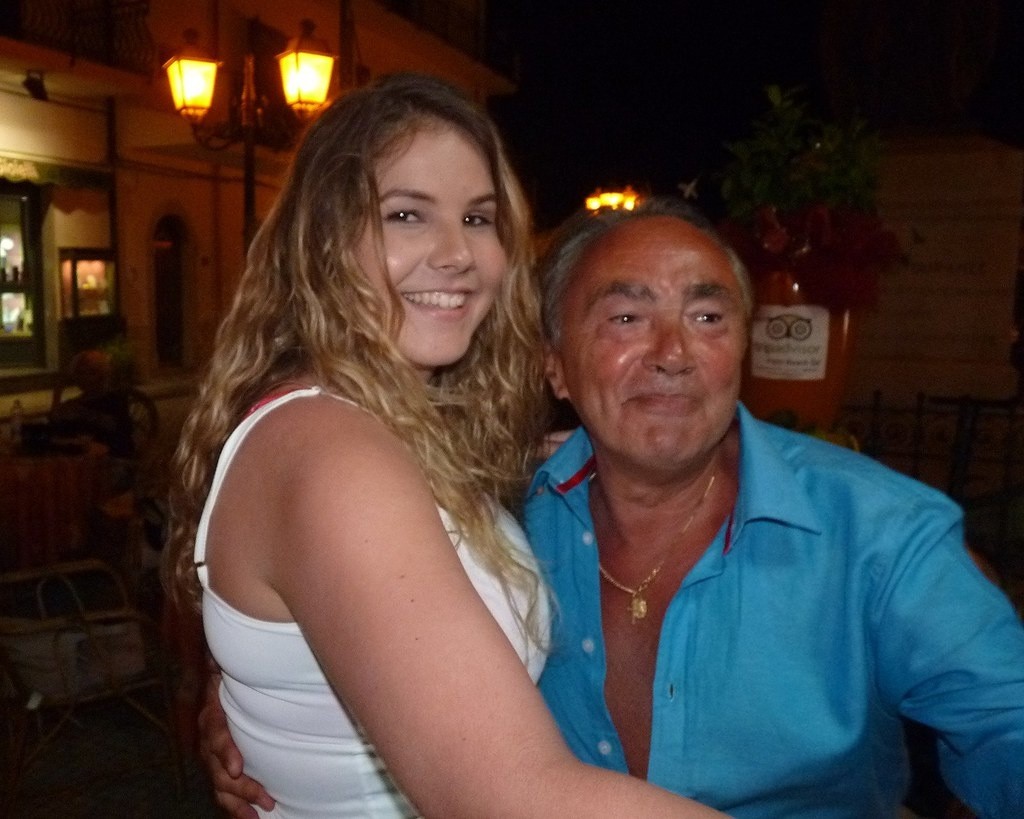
[0,435,108,574]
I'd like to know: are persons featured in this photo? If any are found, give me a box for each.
[162,72,737,819]
[197,199,1024,819]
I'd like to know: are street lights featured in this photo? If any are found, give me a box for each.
[160,21,342,272]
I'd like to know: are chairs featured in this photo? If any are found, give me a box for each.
[117,386,161,524]
[0,557,187,819]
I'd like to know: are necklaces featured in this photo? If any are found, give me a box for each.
[598,476,717,624]
